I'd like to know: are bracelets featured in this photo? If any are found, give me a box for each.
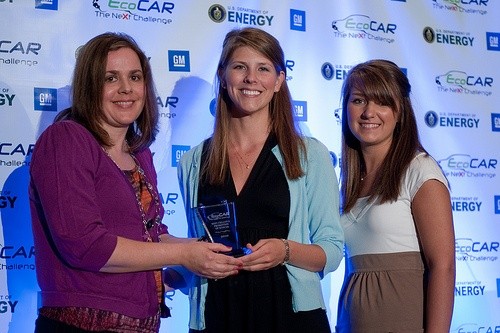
[281,239,289,266]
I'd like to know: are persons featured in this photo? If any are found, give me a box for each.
[334,60,456,333]
[178,29,345,333]
[28,32,242,333]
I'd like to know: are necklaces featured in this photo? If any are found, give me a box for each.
[227,134,262,168]
[360,157,383,180]
